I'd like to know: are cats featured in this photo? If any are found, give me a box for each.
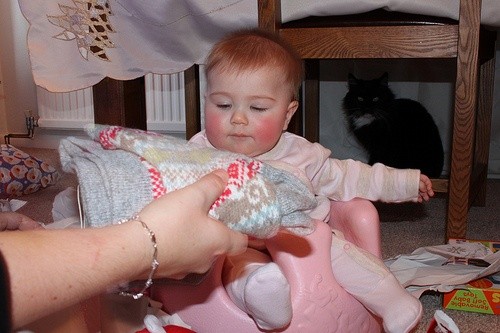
[340,71,445,222]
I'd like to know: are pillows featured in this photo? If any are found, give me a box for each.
[0,144,62,196]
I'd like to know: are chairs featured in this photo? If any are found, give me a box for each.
[256,0,481,245]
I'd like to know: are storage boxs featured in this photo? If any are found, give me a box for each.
[442,238,500,315]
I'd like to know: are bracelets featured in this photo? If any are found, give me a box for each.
[116,218,159,300]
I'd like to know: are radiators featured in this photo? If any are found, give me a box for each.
[36,64,206,135]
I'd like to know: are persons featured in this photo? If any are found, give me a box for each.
[187,29,434,333]
[0,169,248,333]
